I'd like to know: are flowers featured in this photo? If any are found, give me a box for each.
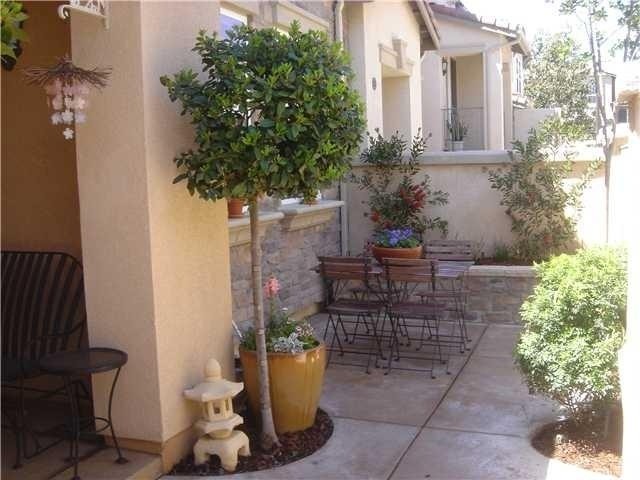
[239,274,319,355]
[371,228,421,248]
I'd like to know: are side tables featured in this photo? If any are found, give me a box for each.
[36,349,131,480]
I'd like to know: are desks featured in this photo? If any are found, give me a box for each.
[308,261,475,365]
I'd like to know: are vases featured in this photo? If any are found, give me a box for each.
[238,343,325,432]
[373,244,423,264]
[228,197,245,217]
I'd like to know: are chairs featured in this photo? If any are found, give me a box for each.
[381,258,448,379]
[412,239,474,354]
[317,255,388,373]
[349,236,407,345]
[0,249,94,471]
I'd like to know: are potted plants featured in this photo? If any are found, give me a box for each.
[446,116,470,151]
